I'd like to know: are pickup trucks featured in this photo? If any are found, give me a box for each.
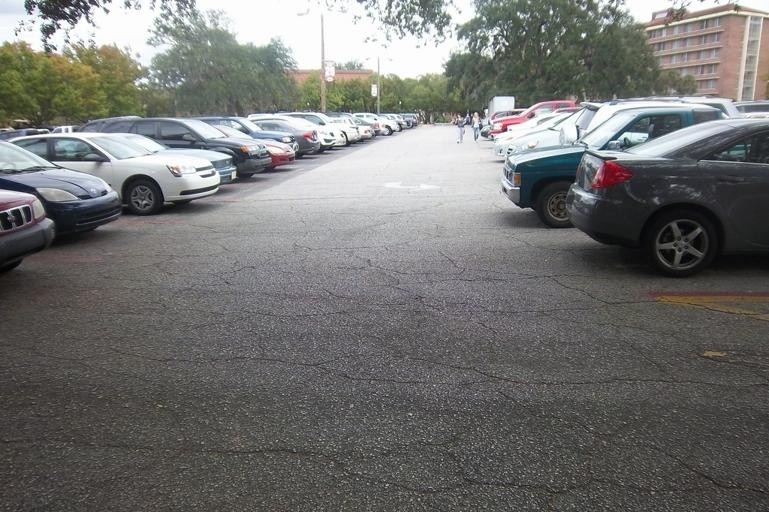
[499,106,725,229]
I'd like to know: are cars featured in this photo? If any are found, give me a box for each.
[7,133,221,216]
[565,115,768,280]
[0,139,123,238]
[0,188,55,275]
[478,96,768,166]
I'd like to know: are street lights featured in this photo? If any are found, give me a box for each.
[294,8,343,111]
[365,54,394,114]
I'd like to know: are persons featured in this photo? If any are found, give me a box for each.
[452,113,466,144]
[472,112,480,142]
[465,114,470,125]
